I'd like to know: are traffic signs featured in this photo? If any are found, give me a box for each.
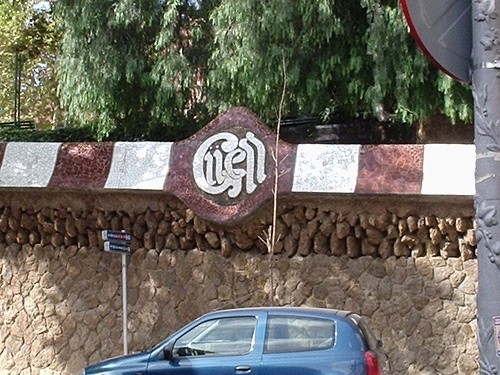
[104,242,131,254]
[101,230,133,242]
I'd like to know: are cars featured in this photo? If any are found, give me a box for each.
[81,307,384,375]
